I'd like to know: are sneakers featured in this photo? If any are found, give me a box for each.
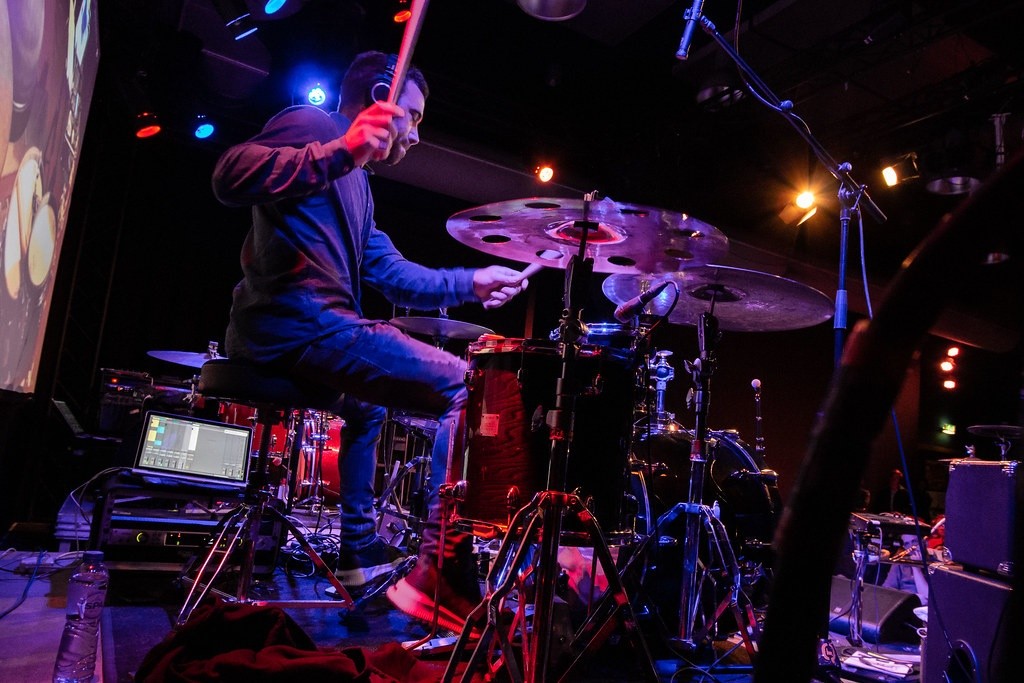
[334,539,405,586]
[387,560,505,640]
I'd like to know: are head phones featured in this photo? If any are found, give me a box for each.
[365,54,399,110]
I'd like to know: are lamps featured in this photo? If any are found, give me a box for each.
[212,0,259,41]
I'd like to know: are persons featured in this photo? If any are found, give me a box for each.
[857,468,907,585]
[215,51,530,643]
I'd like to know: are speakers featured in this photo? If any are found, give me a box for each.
[941,457,1024,582]
[829,575,924,644]
[919,563,1024,683]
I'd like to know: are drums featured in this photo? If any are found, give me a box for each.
[281,407,347,515]
[390,408,442,435]
[464,335,641,548]
[216,400,296,460]
[553,322,655,415]
[620,429,776,642]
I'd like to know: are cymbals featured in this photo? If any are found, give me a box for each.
[600,264,837,332]
[443,194,731,275]
[389,314,496,341]
[147,349,228,369]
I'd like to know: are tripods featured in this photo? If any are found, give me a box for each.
[441,230,761,683]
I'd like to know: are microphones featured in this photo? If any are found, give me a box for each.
[677,0,704,60]
[614,280,668,323]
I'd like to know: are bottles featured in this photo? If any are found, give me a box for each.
[51,550,110,683]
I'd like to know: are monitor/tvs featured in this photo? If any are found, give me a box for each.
[133,409,254,489]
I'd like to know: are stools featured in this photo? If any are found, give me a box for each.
[173,351,367,633]
